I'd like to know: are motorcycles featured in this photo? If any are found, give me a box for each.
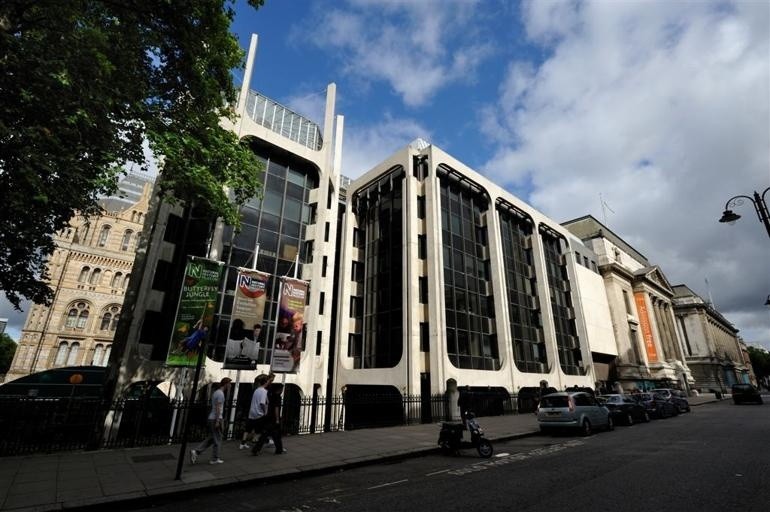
[437,410,493,459]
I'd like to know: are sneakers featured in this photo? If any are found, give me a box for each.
[210,458,223,465]
[240,440,288,456]
[190,449,199,465]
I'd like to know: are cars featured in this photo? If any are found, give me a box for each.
[731,382,763,405]
[537,387,690,436]
[1,365,171,454]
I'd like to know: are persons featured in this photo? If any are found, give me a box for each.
[241,324,261,360]
[288,312,304,362]
[190,377,232,465]
[239,374,287,456]
[442,378,468,430]
[171,320,209,354]
[611,381,623,394]
